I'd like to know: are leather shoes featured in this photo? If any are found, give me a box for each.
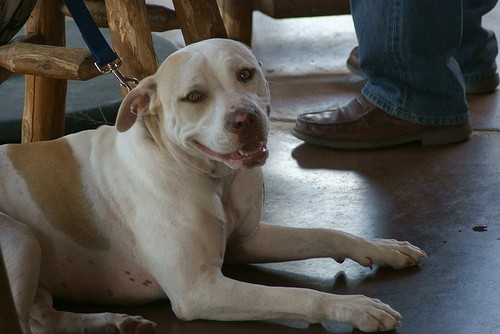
[290,93,472,149]
[346,45,500,93]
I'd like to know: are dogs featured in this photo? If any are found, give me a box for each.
[0,38,429,334]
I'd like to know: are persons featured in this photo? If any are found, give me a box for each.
[289,0,500,150]
